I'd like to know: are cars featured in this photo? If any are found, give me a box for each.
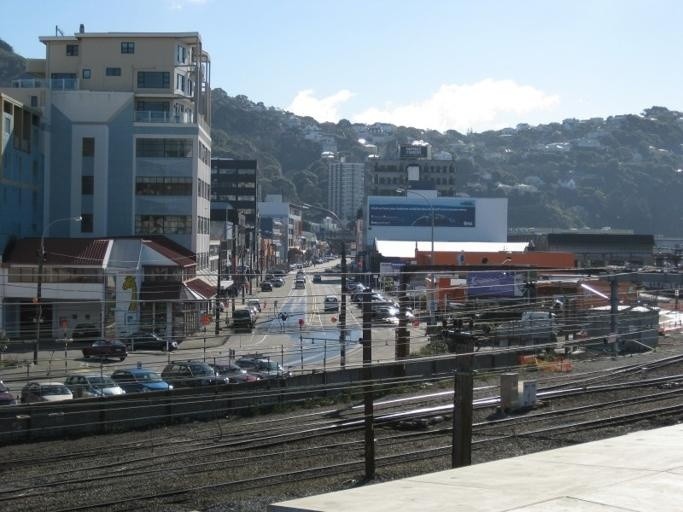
[293,270,307,289]
[1,353,292,406]
[82,330,179,362]
[232,270,285,335]
[344,275,415,326]
[323,296,339,312]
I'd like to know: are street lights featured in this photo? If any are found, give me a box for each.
[394,186,436,323]
[289,199,347,371]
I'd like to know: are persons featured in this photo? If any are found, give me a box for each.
[278,312,282,331]
[280,312,288,331]
